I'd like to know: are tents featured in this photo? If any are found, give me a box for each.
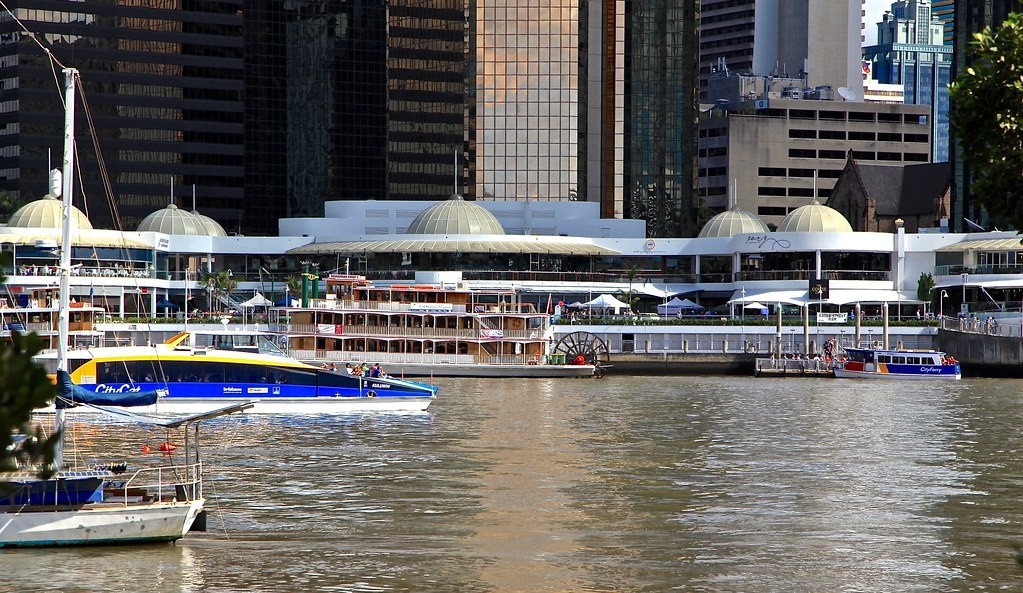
[240,294,275,329]
[568,294,631,315]
[656,298,705,316]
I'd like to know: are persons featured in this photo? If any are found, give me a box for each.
[875,310,879,320]
[783,354,787,369]
[941,356,959,365]
[937,312,941,320]
[19,263,58,275]
[850,310,854,320]
[791,354,809,369]
[114,261,132,276]
[917,309,920,320]
[986,316,997,333]
[111,371,289,384]
[813,337,847,372]
[771,353,774,369]
[687,309,706,320]
[925,311,929,320]
[976,317,981,330]
[861,311,865,320]
[565,309,642,321]
[324,362,387,378]
[677,311,682,320]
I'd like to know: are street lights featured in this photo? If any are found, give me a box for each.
[741,285,746,322]
[209,283,215,320]
[253,287,259,298]
[665,285,669,322]
[819,286,824,325]
[940,290,949,328]
[588,286,592,325]
[285,284,291,324]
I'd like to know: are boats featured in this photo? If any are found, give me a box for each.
[832,340,962,381]
[22,325,439,414]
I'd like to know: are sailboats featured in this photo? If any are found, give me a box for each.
[0,67,263,551]
